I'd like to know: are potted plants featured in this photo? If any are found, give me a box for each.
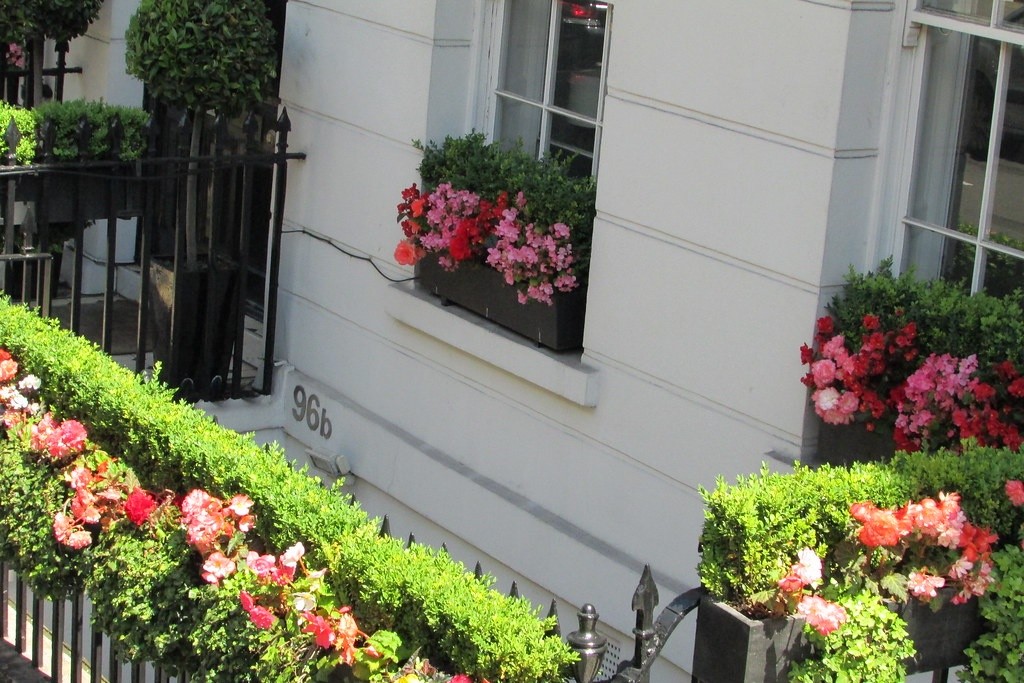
[0,97,149,223]
[121,0,280,402]
[0,0,105,303]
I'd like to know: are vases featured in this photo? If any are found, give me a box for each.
[817,415,897,465]
[691,587,987,682]
[412,245,588,350]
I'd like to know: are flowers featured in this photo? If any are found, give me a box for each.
[393,128,596,306]
[697,442,1024,683]
[800,254,1024,456]
[1,344,478,682]
